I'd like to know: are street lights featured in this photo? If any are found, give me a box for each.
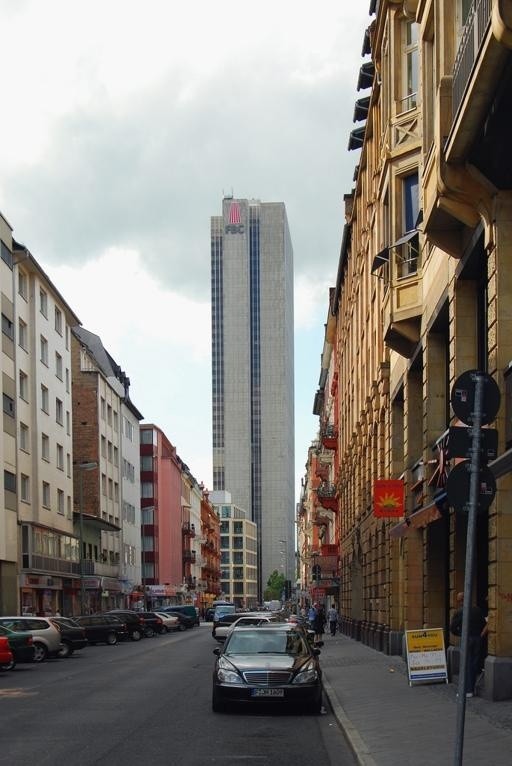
[142,506,156,612]
[79,462,98,615]
[279,540,288,580]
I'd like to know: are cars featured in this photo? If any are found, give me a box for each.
[206,600,324,714]
[0,605,199,671]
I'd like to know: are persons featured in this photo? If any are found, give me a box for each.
[312,609,327,644]
[235,599,317,630]
[55,609,62,616]
[450,591,488,698]
[328,603,339,637]
